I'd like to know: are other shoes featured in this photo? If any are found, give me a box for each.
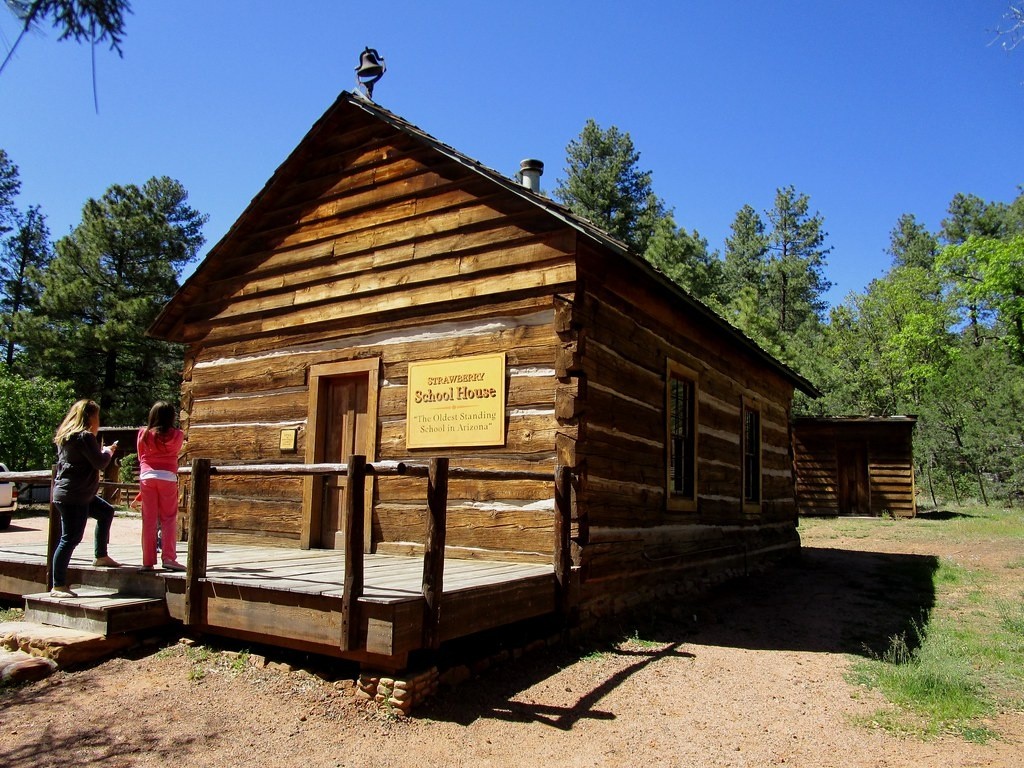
[92,558,122,567]
[142,565,154,571]
[163,560,186,571]
[50,588,78,597]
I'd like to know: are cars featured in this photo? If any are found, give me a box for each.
[0,463,19,530]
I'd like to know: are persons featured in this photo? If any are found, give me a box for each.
[130,401,187,571]
[50,399,122,598]
